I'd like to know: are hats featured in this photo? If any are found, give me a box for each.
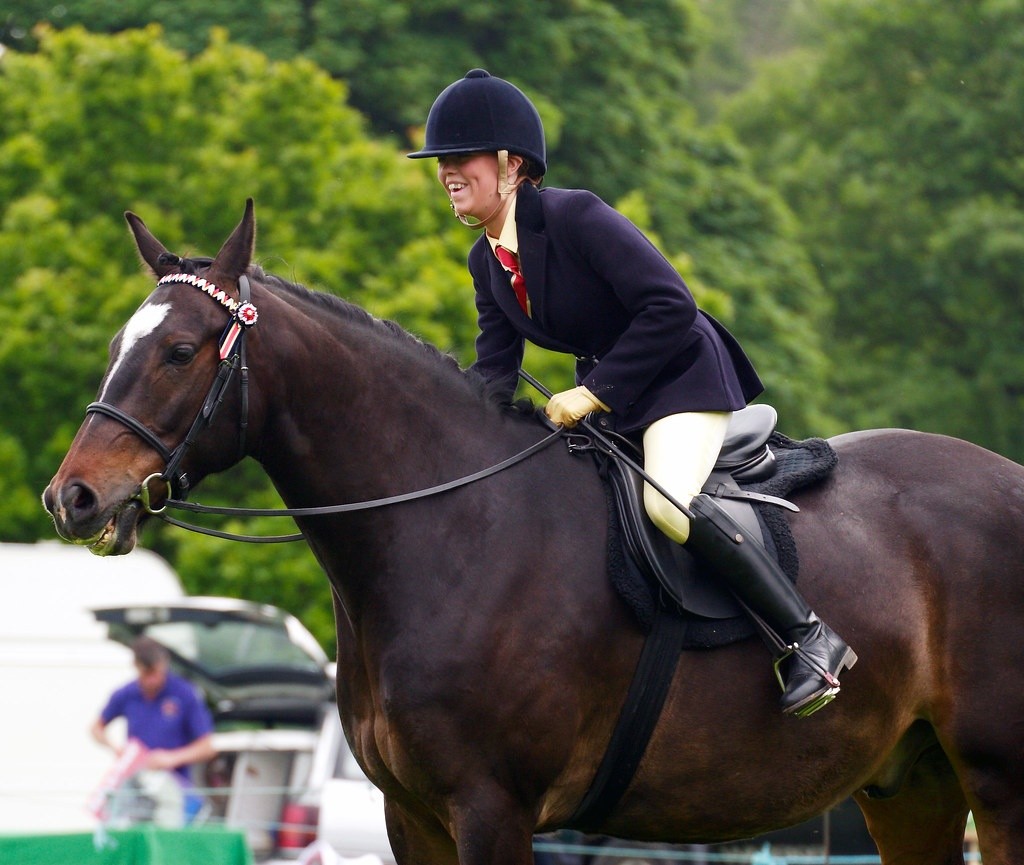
[134,637,163,668]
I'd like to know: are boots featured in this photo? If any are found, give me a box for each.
[680,494,858,717]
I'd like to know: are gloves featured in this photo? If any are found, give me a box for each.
[545,386,602,428]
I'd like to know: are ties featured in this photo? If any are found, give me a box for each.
[496,244,531,320]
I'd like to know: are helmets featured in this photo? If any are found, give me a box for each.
[406,69,548,176]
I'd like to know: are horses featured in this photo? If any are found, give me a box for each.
[42,197,1024,865]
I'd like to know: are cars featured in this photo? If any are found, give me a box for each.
[85,598,398,865]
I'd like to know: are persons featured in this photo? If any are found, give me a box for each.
[91,639,216,828]
[407,69,858,718]
